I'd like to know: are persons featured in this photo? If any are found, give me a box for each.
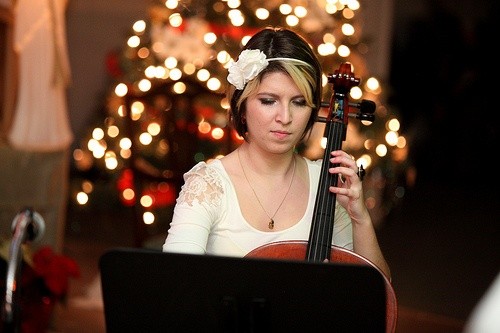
[162,29,392,284]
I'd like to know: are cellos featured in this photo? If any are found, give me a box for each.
[243,64,398,333]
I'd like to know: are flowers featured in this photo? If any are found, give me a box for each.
[227,49,268,91]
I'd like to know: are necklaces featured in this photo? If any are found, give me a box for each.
[235,142,297,230]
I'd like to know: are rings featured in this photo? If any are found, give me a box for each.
[356,165,367,181]
